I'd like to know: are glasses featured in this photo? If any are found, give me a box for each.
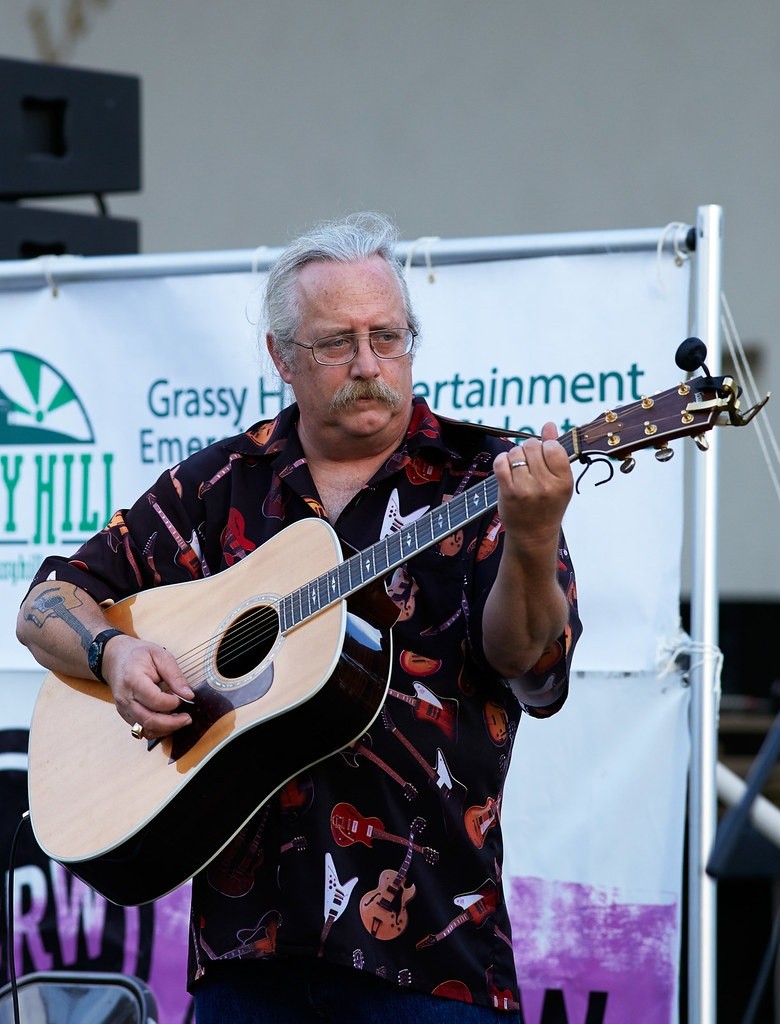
[287,320,419,366]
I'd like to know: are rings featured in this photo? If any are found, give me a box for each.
[131,723,143,739]
[511,461,527,469]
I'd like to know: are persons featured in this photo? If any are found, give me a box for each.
[15,211,584,1024]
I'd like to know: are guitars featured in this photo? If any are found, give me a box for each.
[25,336,773,909]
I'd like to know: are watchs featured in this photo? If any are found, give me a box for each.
[88,628,126,685]
